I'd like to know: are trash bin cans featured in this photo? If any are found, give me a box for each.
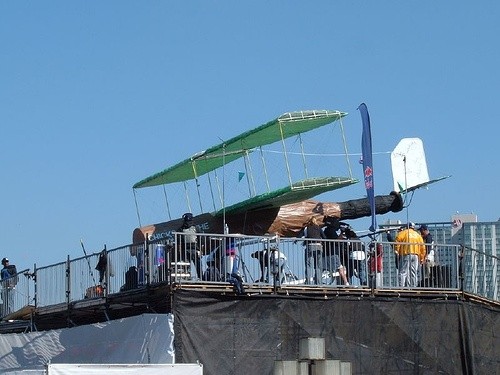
[429,263,452,286]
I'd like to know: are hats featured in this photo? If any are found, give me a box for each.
[2,258,8,264]
[418,224,428,230]
[181,213,193,221]
[408,221,415,228]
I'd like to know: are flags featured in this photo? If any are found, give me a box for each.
[356,103,378,232]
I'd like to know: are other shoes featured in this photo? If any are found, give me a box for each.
[345,282,349,285]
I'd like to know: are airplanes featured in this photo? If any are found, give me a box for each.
[130,110,452,271]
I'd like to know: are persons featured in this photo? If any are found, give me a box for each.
[1,257,18,318]
[294,218,326,284]
[324,227,368,289]
[251,250,286,286]
[394,222,426,287]
[386,227,405,269]
[416,224,436,281]
[174,213,204,280]
